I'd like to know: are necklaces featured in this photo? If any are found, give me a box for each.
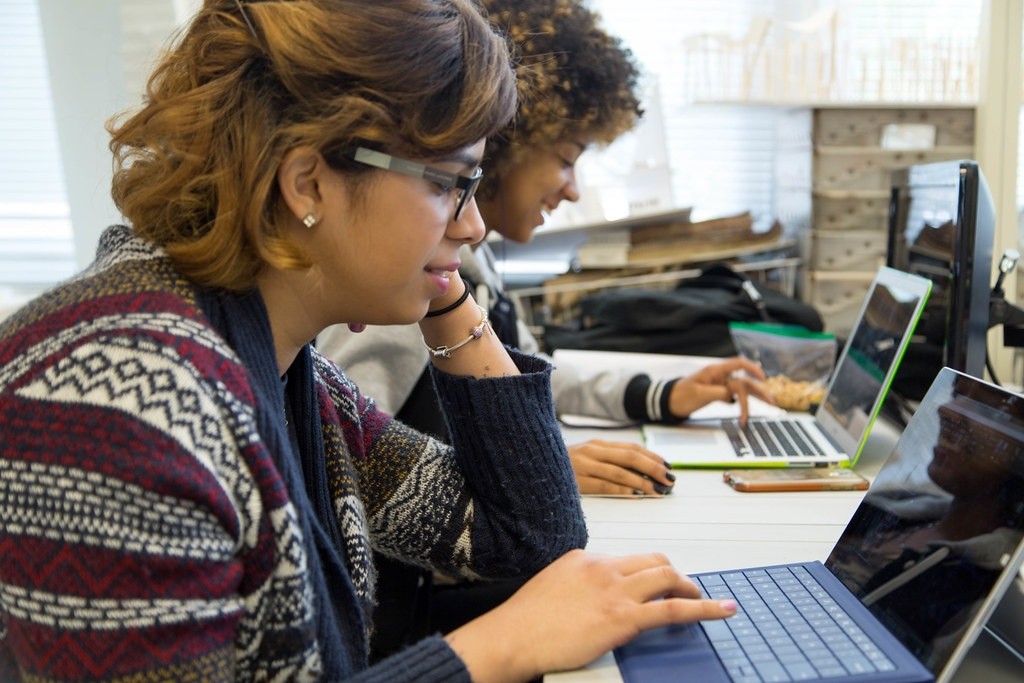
[898,520,941,549]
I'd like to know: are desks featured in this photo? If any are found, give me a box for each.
[536,340,1024,683]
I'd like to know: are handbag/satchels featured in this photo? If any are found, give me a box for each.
[544,262,825,362]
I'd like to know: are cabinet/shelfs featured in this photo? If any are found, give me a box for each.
[800,101,977,341]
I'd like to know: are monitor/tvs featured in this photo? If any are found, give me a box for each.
[881,158,992,401]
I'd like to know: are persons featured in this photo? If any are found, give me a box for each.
[836,376,1024,654]
[1,0,736,683]
[312,0,775,666]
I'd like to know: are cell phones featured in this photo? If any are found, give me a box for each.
[723,468,869,493]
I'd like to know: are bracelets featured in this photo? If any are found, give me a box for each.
[425,278,470,318]
[422,305,488,357]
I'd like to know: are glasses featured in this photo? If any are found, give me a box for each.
[329,143,482,223]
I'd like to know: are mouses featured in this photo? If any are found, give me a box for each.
[620,463,674,496]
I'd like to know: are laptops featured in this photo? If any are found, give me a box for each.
[614,367,1024,683]
[639,262,932,469]
